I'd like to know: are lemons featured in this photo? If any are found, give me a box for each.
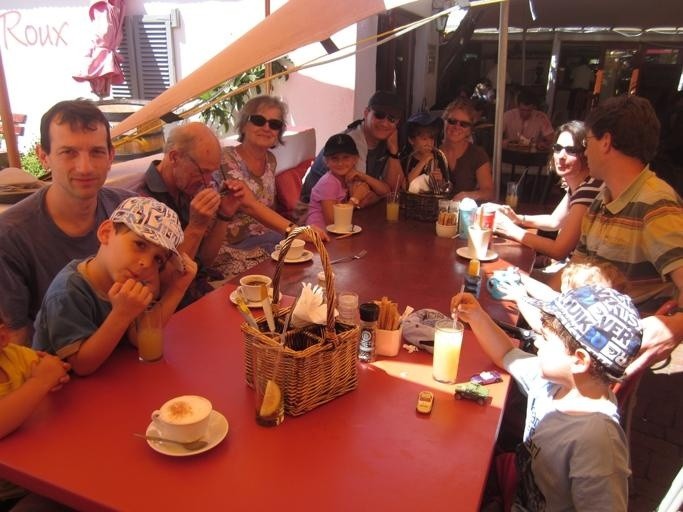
[259,381,281,417]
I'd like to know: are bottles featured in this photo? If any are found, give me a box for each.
[317,270,376,368]
[438,198,459,215]
[414,392,434,415]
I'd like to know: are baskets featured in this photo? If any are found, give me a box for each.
[397,143,455,225]
[239,225,363,422]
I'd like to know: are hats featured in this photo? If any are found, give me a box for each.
[321,133,360,157]
[108,193,189,279]
[367,88,405,120]
[400,109,444,149]
[523,278,645,379]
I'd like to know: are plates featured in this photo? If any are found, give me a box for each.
[519,147,537,152]
[229,288,282,306]
[455,246,500,263]
[326,222,364,235]
[507,138,539,147]
[270,246,313,263]
[144,409,229,458]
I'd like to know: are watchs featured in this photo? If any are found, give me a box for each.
[281,223,295,240]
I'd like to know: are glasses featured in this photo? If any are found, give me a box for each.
[552,141,581,156]
[369,110,397,124]
[244,113,284,130]
[446,116,472,129]
[182,151,230,201]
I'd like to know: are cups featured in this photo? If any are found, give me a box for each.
[148,394,212,442]
[275,237,304,258]
[433,320,464,385]
[458,206,496,241]
[333,204,354,231]
[238,272,272,300]
[250,331,285,427]
[467,225,492,258]
[387,192,399,223]
[133,299,166,365]
[375,324,403,358]
[504,181,520,208]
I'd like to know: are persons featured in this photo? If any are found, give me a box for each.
[129,123,244,311]
[0,98,141,350]
[297,91,491,233]
[31,197,199,377]
[479,89,683,413]
[0,317,69,439]
[205,96,330,285]
[445,284,645,512]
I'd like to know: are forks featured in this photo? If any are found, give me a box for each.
[325,248,368,265]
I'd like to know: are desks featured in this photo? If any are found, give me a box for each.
[501,139,549,199]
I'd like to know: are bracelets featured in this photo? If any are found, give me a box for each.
[216,208,233,223]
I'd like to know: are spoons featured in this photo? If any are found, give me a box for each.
[131,430,206,451]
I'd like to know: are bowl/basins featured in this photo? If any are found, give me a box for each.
[436,220,458,239]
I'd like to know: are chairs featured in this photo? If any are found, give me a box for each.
[609,331,682,414]
[275,159,313,215]
